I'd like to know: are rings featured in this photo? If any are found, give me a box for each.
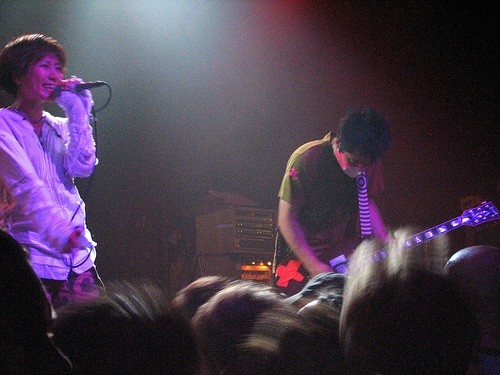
[70,76,77,78]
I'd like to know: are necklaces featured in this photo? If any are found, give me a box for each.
[17,109,46,124]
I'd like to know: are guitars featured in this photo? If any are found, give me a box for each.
[273,199,500,297]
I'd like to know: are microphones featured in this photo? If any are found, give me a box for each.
[54,81,106,97]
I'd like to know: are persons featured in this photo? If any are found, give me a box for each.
[0,226,500,375]
[0,34,103,310]
[272,111,393,295]
